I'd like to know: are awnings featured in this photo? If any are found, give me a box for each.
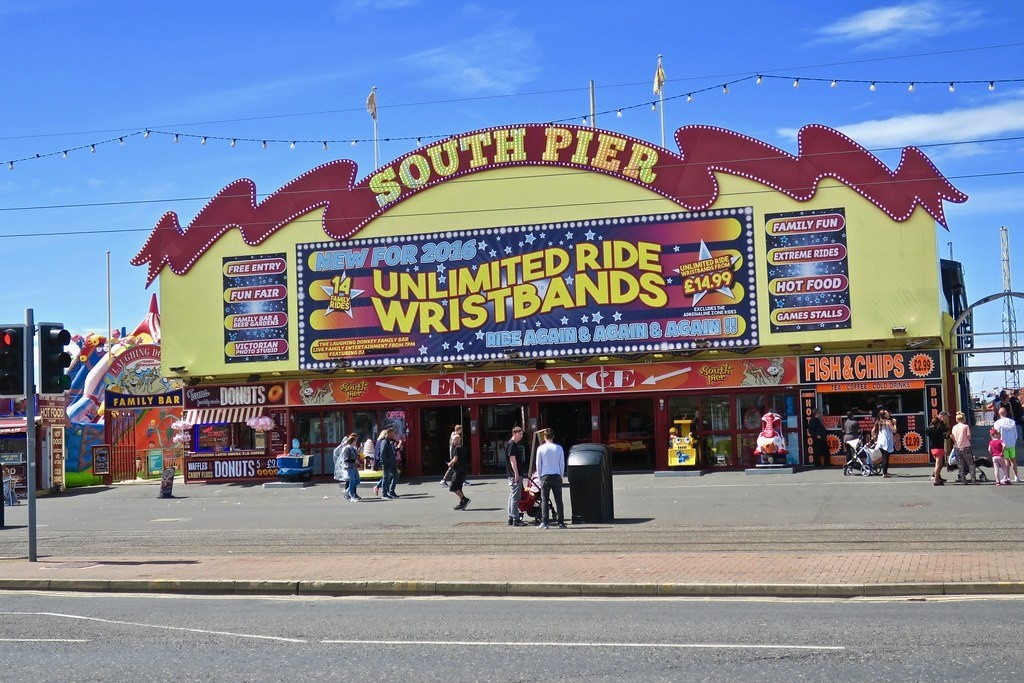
[186,407,263,426]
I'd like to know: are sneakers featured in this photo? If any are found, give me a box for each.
[507,517,513,525]
[559,523,567,529]
[461,497,471,510]
[453,501,463,510]
[373,486,378,495]
[439,479,450,487]
[537,523,548,530]
[388,490,399,498]
[463,481,471,486]
[513,519,527,526]
[382,493,393,500]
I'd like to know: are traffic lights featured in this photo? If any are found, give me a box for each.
[0,323,26,398]
[38,322,73,393]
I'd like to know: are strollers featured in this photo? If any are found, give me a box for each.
[843,438,882,477]
[517,475,559,525]
[946,436,993,482]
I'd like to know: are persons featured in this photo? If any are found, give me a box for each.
[844,412,863,475]
[332,432,361,502]
[808,409,834,468]
[505,426,528,527]
[536,428,568,530]
[949,411,978,485]
[872,409,897,478]
[988,427,1010,485]
[525,478,542,497]
[994,408,1021,482]
[372,425,401,501]
[440,424,472,488]
[988,389,1024,446]
[925,411,951,486]
[446,435,471,511]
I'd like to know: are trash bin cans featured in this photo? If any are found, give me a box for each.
[567,443,614,524]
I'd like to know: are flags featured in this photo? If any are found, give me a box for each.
[366,93,378,121]
[653,64,666,95]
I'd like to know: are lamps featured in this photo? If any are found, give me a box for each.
[169,366,188,372]
[891,326,907,335]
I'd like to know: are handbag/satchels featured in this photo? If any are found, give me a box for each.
[949,448,958,466]
[866,446,882,460]
[947,463,958,472]
[951,457,957,465]
[950,450,957,463]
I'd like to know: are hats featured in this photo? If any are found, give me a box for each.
[990,428,996,436]
[940,411,951,417]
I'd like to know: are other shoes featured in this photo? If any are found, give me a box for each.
[931,472,1021,486]
[823,463,834,467]
[882,475,891,478]
[343,489,361,503]
[815,464,824,468]
